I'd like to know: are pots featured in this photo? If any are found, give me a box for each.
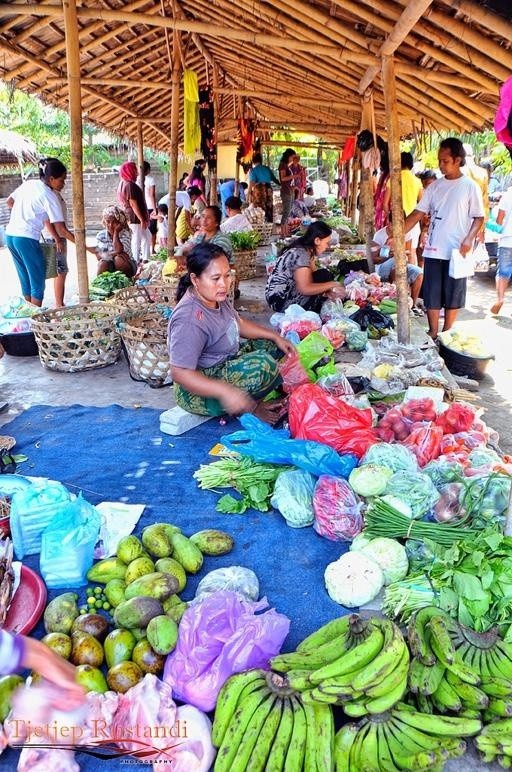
[0,474,34,541]
[437,335,496,382]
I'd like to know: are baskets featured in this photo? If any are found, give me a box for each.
[110,282,180,313]
[116,303,180,390]
[231,249,258,281]
[28,299,126,375]
[250,221,275,246]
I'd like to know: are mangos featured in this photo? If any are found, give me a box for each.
[0,522,236,726]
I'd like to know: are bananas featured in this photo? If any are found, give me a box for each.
[211,605,512,772]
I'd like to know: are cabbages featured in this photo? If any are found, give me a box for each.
[324,531,410,608]
[348,463,386,497]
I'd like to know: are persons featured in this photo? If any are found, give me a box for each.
[37,156,71,309]
[164,242,296,426]
[4,162,99,309]
[95,134,501,340]
[489,186,511,315]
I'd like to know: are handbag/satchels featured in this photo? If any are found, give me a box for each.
[37,241,61,281]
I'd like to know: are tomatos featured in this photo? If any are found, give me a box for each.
[378,398,437,442]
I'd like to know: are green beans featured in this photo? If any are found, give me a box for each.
[363,470,500,549]
